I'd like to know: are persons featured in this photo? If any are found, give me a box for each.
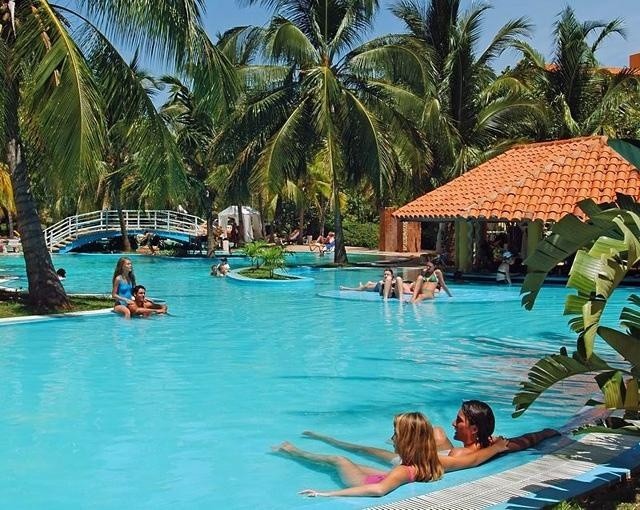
[272,400,562,496]
[143,231,154,254]
[340,259,453,304]
[496,256,515,287]
[57,269,66,281]
[211,258,229,278]
[112,257,167,320]
[316,232,335,244]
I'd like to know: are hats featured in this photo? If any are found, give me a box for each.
[502,251,513,258]
